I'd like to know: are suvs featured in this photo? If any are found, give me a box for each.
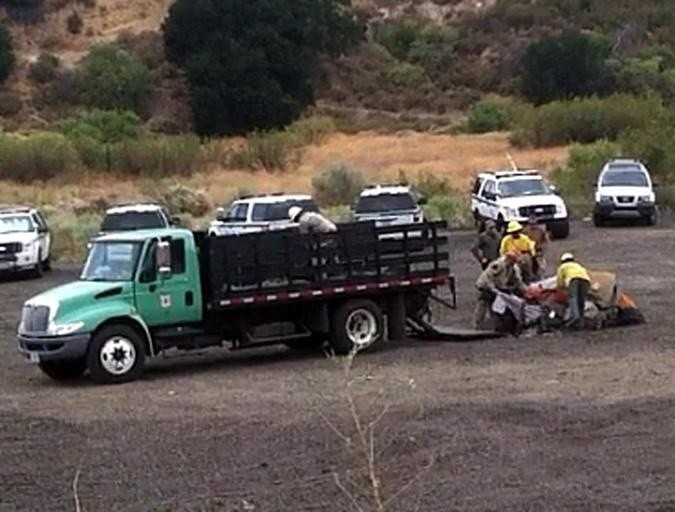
[206,191,319,237]
[94,204,179,265]
[0,208,55,279]
[470,152,570,242]
[591,156,660,227]
[352,183,437,250]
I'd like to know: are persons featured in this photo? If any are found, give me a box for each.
[470,213,648,331]
[288,205,338,280]
[196,207,227,232]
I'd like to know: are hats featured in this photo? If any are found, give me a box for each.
[506,248,521,262]
[561,252,574,261]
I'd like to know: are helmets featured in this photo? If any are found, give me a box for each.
[506,220,524,233]
[287,206,303,223]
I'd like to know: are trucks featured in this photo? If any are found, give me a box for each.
[15,217,451,385]
